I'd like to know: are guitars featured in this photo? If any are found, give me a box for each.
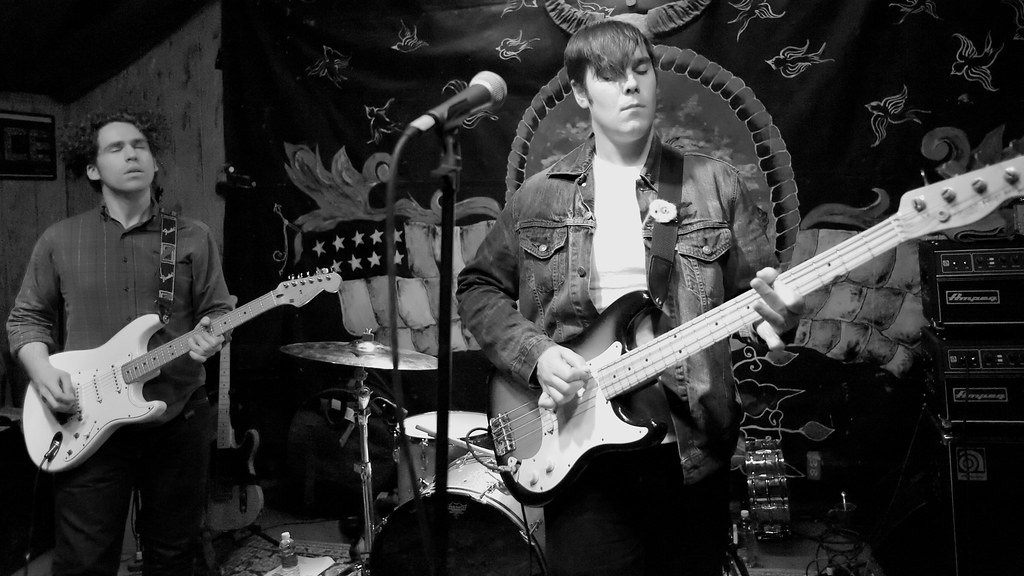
[22,268,342,473]
[484,155,1024,509]
[205,294,265,534]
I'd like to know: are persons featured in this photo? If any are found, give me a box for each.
[455,22,804,576]
[6,109,232,576]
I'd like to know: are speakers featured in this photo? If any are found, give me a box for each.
[912,403,1024,576]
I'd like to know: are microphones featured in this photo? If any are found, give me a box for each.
[422,70,509,131]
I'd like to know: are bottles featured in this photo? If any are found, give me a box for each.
[279,531,300,576]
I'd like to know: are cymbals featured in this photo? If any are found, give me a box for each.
[278,333,438,372]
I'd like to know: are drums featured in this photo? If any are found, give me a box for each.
[744,438,792,541]
[394,410,492,504]
[369,453,548,575]
